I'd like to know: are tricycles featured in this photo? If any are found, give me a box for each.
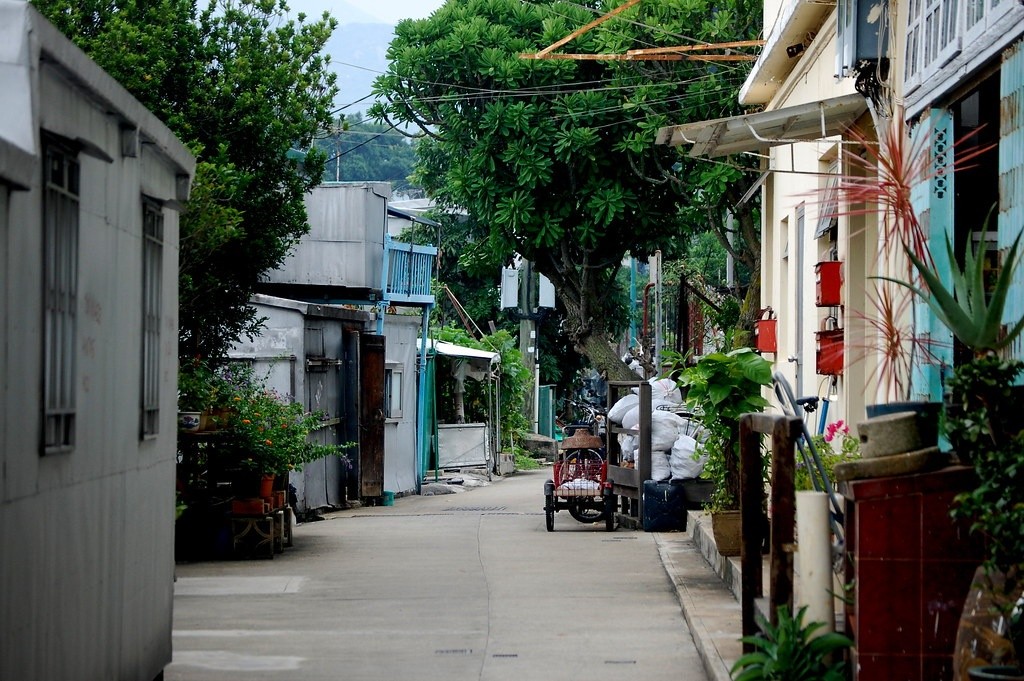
[544,427,615,531]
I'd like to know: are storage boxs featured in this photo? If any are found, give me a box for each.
[814,261,843,307]
[754,319,777,354]
[814,327,844,376]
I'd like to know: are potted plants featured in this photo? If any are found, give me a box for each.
[177,353,359,516]
[654,343,776,558]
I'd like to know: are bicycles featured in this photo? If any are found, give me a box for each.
[544,399,607,499]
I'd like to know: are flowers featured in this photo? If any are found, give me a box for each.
[794,420,861,494]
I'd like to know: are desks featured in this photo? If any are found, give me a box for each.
[225,503,293,556]
